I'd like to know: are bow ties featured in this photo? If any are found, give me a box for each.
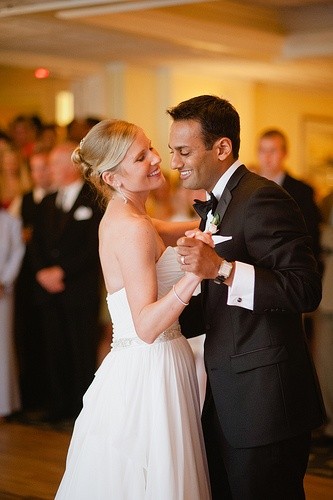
[192,191,218,220]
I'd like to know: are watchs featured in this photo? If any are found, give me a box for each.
[213,258,234,285]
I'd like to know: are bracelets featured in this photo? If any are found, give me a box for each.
[172,284,189,306]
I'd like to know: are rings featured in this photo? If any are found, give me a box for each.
[181,256,186,264]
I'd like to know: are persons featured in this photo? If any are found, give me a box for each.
[1,111,322,426]
[53,118,213,500]
[166,94,329,500]
